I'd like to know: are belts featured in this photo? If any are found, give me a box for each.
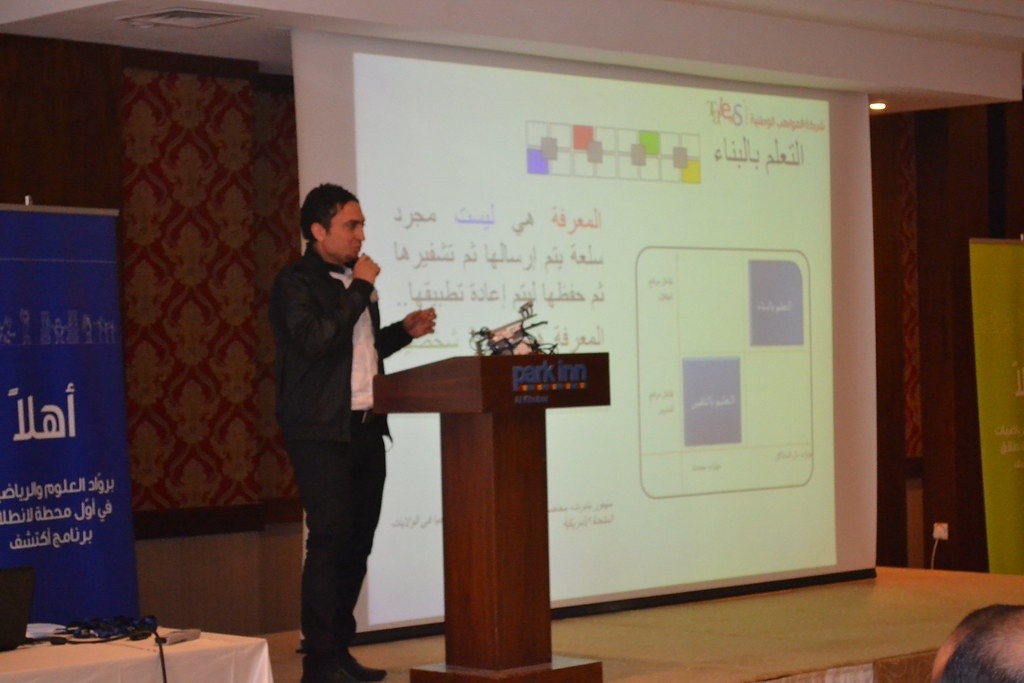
[354,411,379,426]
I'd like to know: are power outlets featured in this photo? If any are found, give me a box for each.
[933,523,949,540]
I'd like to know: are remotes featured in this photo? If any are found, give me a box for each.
[155,628,202,645]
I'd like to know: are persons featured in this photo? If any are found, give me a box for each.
[267,182,437,683]
[928,603,1024,683]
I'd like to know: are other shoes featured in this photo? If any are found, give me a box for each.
[335,649,387,683]
[299,657,358,683]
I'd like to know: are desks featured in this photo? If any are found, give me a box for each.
[0,625,274,683]
